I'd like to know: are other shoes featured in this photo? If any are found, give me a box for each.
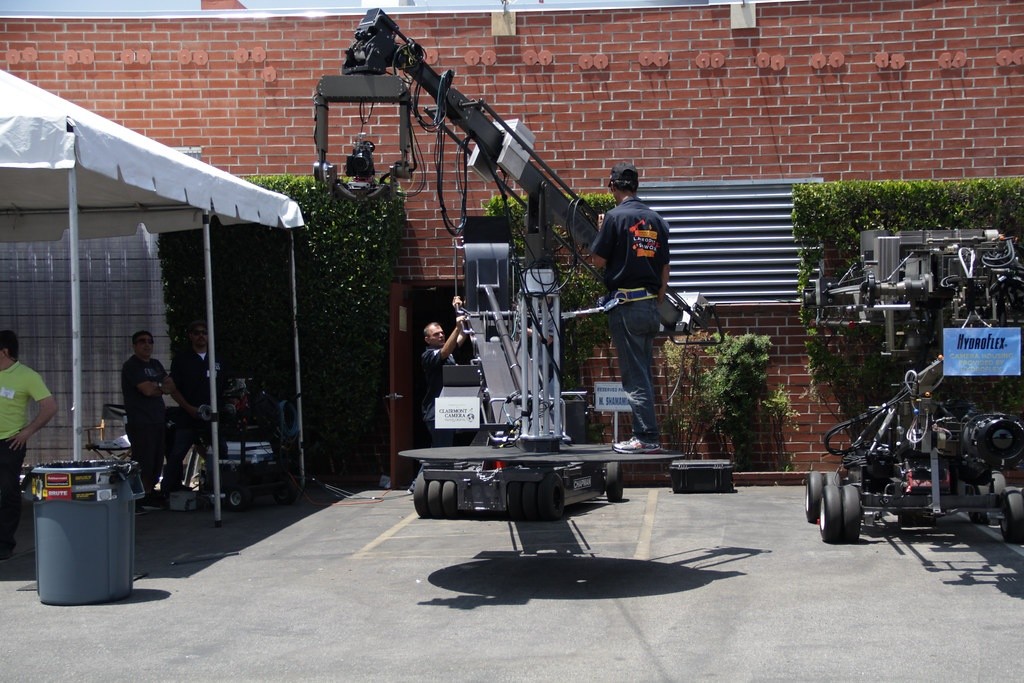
[137,494,167,509]
[0,550,13,559]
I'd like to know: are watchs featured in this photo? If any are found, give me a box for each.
[158,381,162,389]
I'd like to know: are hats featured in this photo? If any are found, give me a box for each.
[608,162,638,187]
[191,320,206,329]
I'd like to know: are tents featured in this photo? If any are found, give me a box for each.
[0,65,307,529]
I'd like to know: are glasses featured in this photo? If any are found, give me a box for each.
[135,339,154,345]
[192,330,207,335]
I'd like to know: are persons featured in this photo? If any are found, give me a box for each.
[121,318,177,513]
[590,162,671,454]
[528,297,554,357]
[407,296,468,495]
[165,319,229,491]
[0,328,60,559]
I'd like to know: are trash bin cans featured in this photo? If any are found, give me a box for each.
[31,459,146,605]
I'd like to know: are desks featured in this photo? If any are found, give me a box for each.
[86,441,132,461]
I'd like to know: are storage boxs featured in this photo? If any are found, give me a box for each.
[667,459,736,493]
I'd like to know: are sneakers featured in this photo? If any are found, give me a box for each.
[611,436,661,454]
